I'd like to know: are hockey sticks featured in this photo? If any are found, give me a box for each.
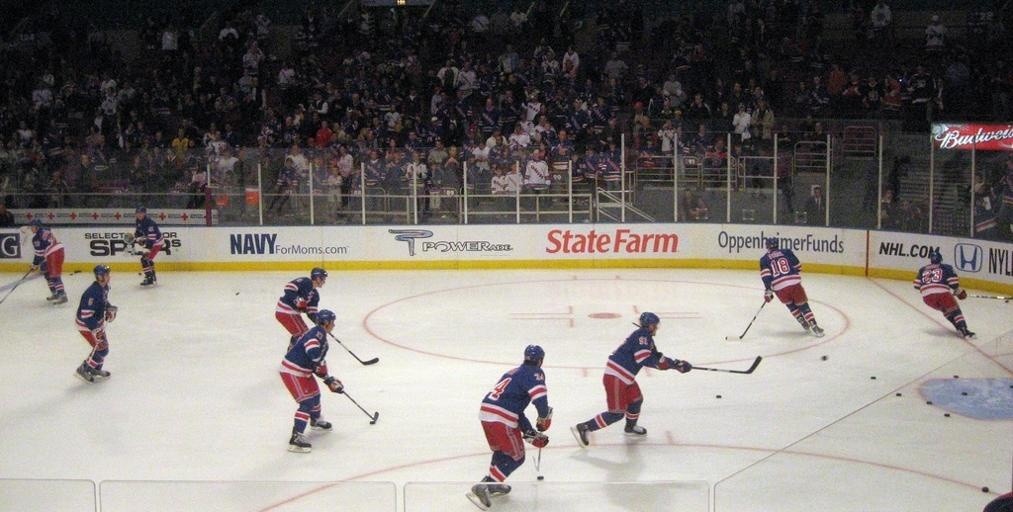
[692,356,762,374]
[739,296,774,339]
[341,390,379,425]
[329,332,379,366]
[533,447,544,479]
[83,314,111,375]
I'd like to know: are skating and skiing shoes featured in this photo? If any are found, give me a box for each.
[957,329,969,337]
[570,423,590,448]
[465,484,491,512]
[288,430,312,453]
[812,325,826,337]
[73,361,95,385]
[140,272,154,287]
[153,270,157,284]
[92,370,111,380]
[47,293,59,302]
[488,481,511,498]
[52,294,68,307]
[964,331,975,339]
[624,422,647,437]
[310,420,332,433]
[804,325,812,335]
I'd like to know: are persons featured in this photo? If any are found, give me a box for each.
[914,251,976,341]
[0,0,169,207]
[75,265,117,385]
[276,266,329,355]
[277,309,344,454]
[166,0,1013,240]
[29,218,69,306]
[133,204,165,288]
[570,313,693,448]
[464,344,553,512]
[758,236,825,337]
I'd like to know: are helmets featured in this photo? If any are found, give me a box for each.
[640,312,660,333]
[94,264,111,281]
[316,309,336,325]
[31,220,42,226]
[136,206,147,214]
[312,268,328,280]
[524,345,544,361]
[931,252,942,263]
[766,236,778,249]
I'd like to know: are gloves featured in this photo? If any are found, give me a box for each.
[96,329,105,341]
[536,416,551,432]
[106,307,117,323]
[676,361,691,372]
[525,429,549,447]
[764,289,772,303]
[92,329,107,344]
[659,356,673,369]
[957,290,966,300]
[323,376,344,393]
[296,298,307,313]
